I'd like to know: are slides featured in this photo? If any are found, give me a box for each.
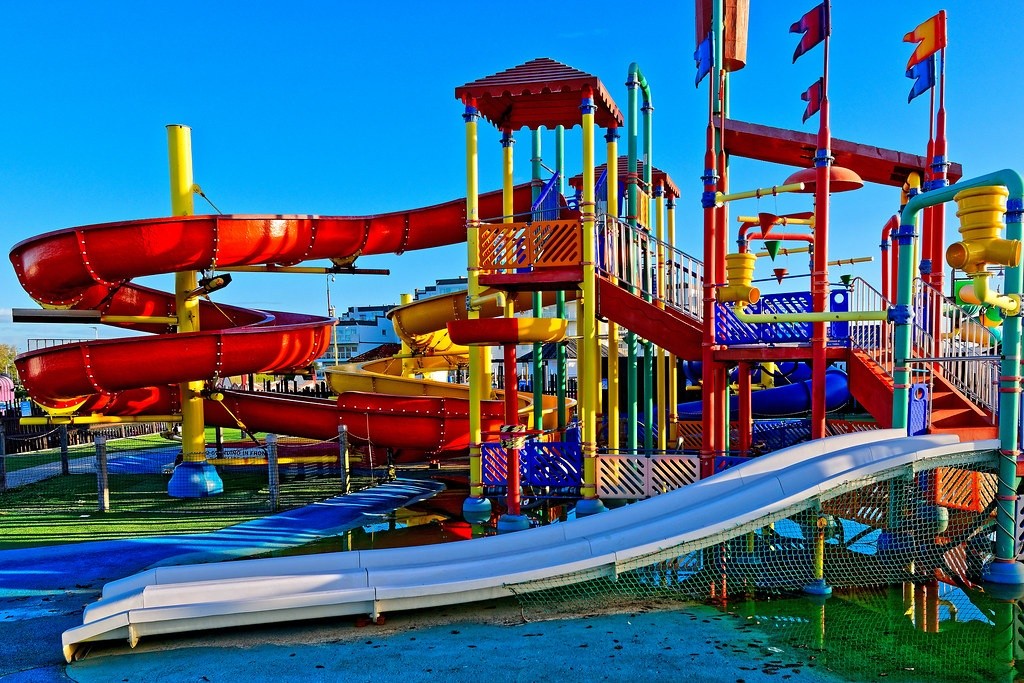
[7,176,1001,665]
